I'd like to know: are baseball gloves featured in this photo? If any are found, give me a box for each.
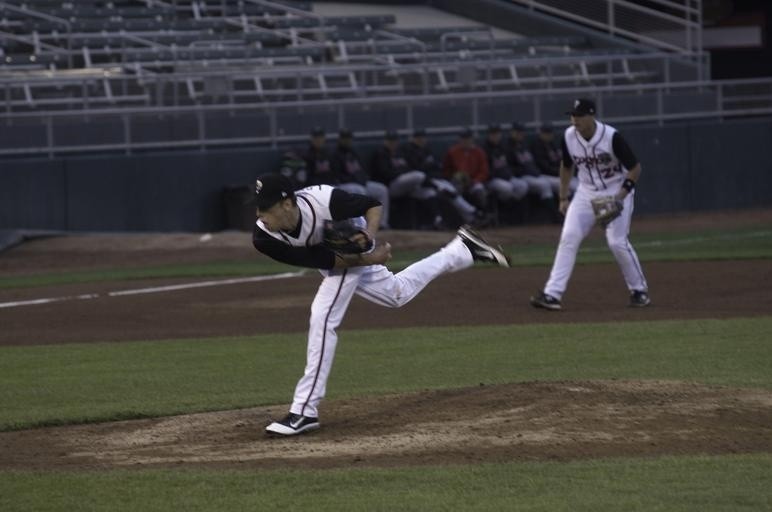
[453,169,472,192]
[591,194,623,225]
[321,220,372,254]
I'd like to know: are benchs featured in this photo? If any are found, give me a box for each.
[391,189,557,230]
[0,0,711,115]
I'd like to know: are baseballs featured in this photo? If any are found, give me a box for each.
[199,233,212,241]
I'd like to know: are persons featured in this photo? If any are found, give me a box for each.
[250,172,512,438]
[530,98,651,311]
[278,120,579,231]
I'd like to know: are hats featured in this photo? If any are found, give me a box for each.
[565,98,595,115]
[245,172,292,211]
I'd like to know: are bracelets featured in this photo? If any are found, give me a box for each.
[622,179,635,192]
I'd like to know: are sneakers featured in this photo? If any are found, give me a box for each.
[266,413,319,436]
[530,291,561,310]
[629,290,650,308]
[456,223,510,267]
